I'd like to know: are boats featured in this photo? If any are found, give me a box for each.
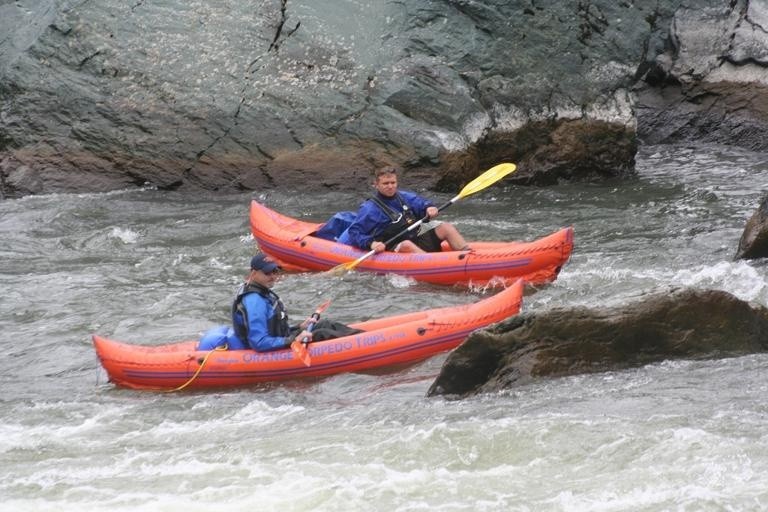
[245,194,579,293]
[87,273,534,402]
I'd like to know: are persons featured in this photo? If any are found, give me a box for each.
[350,165,472,255]
[229,252,367,352]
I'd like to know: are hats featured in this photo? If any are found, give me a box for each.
[250,253,281,273]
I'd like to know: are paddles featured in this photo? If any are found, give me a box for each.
[330,163,518,272]
[289,301,331,367]
